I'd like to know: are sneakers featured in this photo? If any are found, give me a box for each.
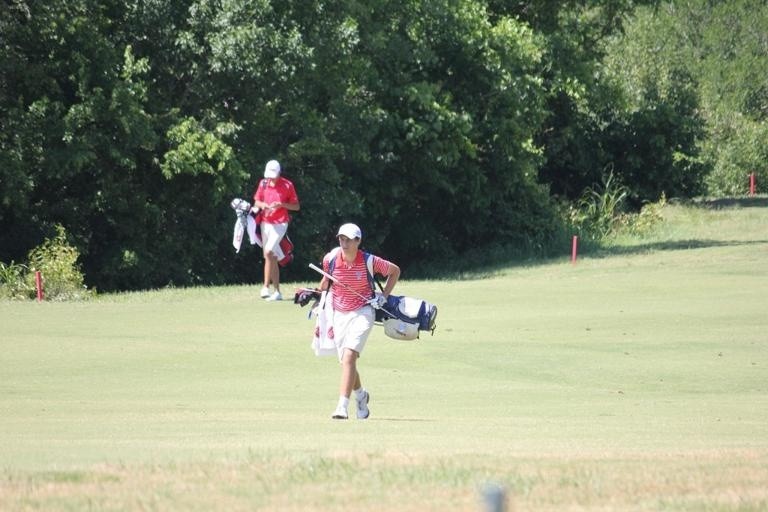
[332,406,348,418]
[261,287,282,301]
[356,392,369,418]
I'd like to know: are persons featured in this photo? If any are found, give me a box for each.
[250,158,300,302]
[312,222,402,422]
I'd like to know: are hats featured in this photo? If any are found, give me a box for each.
[336,223,362,240]
[264,160,280,178]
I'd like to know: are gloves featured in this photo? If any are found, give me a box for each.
[366,295,387,309]
